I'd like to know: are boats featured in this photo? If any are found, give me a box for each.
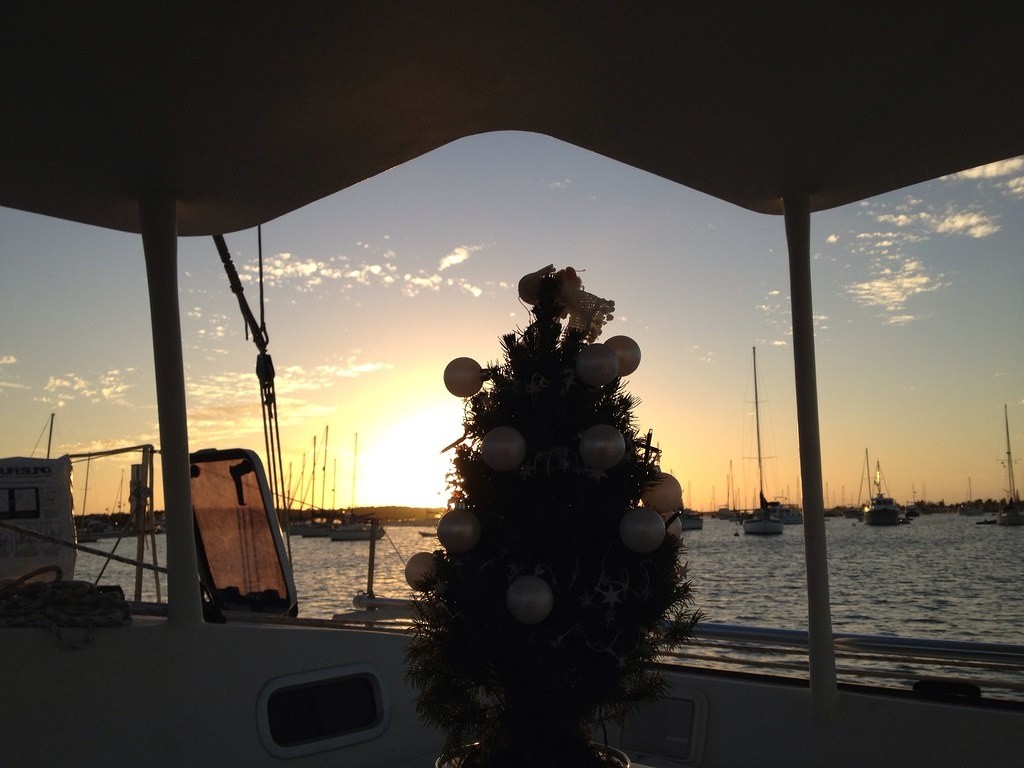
[976,519,997,525]
[76,450,166,542]
[0,454,77,582]
[679,510,704,529]
[417,530,438,537]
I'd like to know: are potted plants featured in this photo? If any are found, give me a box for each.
[404,260,700,768]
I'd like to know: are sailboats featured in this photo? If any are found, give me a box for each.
[958,477,984,516]
[278,426,386,541]
[996,404,1024,526]
[824,446,957,526]
[712,347,803,535]
